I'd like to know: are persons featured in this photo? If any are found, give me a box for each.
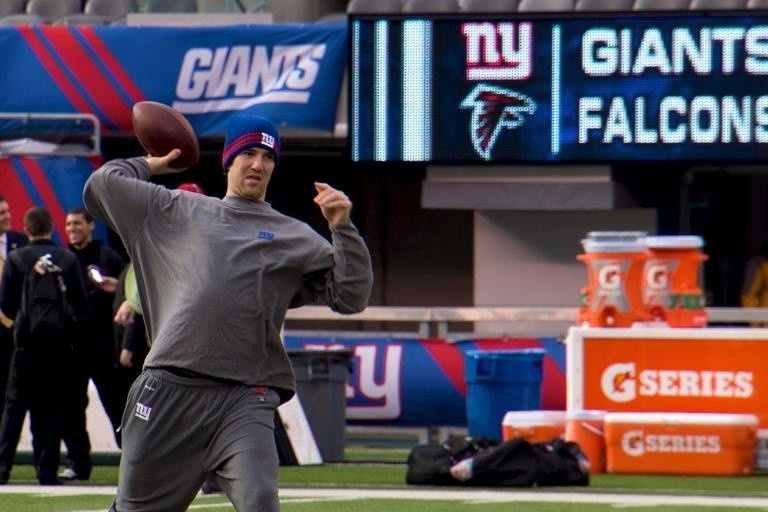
[82,114,373,511]
[0,195,48,478]
[0,205,84,483]
[113,183,222,494]
[55,208,132,479]
[740,261,767,326]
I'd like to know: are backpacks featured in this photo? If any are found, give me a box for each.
[14,247,79,352]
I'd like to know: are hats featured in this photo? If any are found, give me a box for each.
[177,183,204,194]
[222,114,281,172]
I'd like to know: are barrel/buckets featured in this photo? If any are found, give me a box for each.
[272,349,352,463]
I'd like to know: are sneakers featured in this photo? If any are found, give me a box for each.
[58,468,77,480]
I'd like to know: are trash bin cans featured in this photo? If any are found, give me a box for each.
[284,349,354,463]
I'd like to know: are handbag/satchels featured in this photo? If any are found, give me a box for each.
[406,433,589,487]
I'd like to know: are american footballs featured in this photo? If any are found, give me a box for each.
[133,102,199,168]
